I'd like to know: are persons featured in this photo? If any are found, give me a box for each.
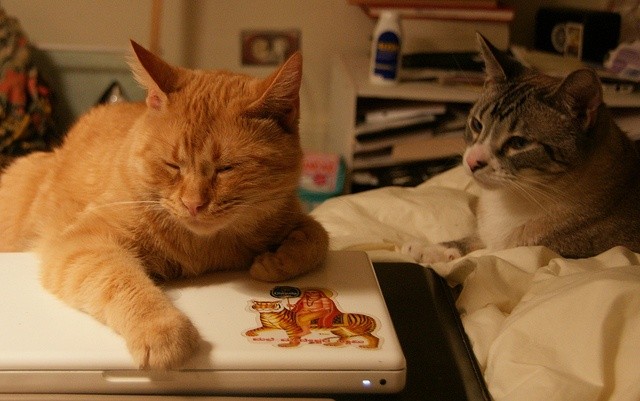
[286,288,341,338]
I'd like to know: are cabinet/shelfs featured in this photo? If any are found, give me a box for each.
[327,58,640,196]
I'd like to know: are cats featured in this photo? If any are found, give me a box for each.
[0,37,332,371]
[398,30,639,265]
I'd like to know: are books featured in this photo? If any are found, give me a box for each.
[350,104,470,192]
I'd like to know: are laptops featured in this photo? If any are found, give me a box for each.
[0,249,408,393]
